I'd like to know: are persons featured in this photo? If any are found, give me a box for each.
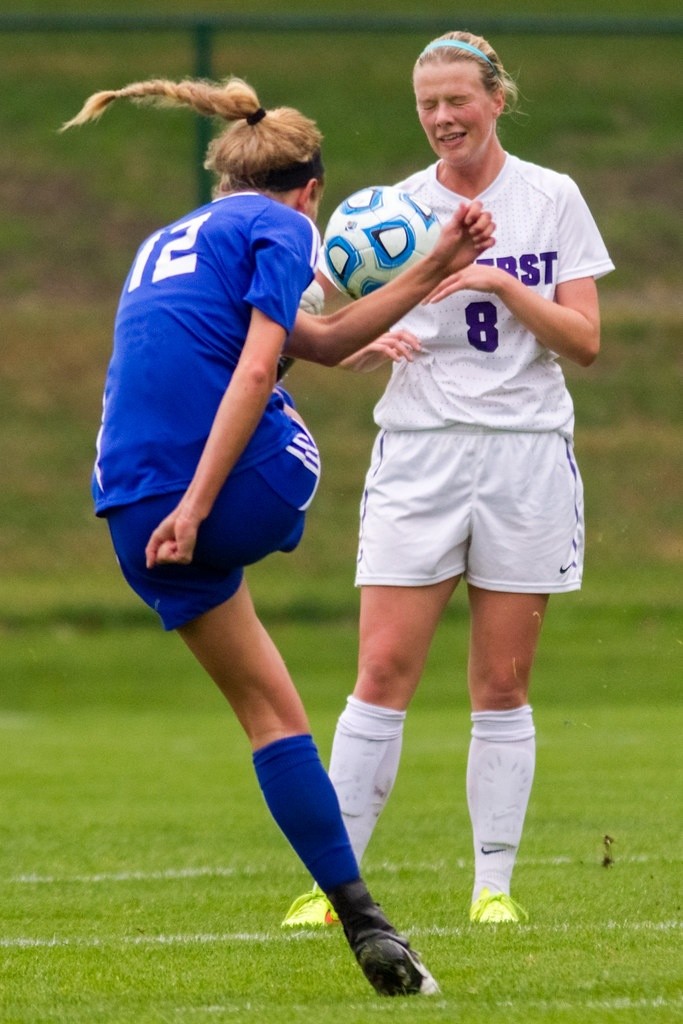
[65,78,496,998]
[280,32,615,927]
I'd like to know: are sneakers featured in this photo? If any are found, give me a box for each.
[281,884,342,926]
[470,886,528,924]
[341,904,442,995]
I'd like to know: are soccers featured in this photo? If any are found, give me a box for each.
[322,184,444,301]
